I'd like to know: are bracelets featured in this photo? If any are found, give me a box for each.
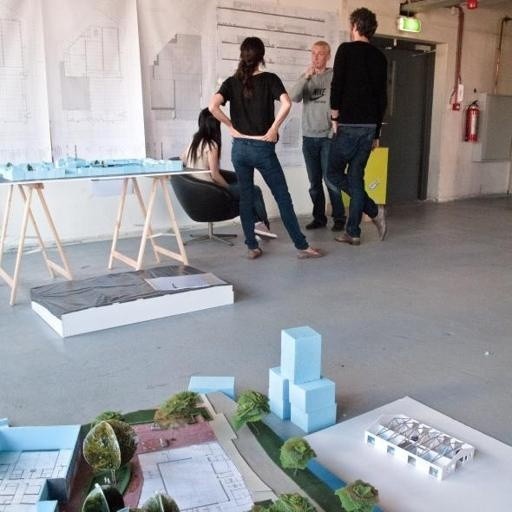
[330,115,339,121]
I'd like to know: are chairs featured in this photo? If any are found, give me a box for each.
[169,157,238,247]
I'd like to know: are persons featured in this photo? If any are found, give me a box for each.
[182,106,277,238]
[287,40,347,232]
[207,35,323,260]
[324,6,389,247]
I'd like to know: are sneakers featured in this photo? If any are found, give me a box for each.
[306,219,326,230]
[331,222,345,232]
[249,248,262,258]
[254,223,278,239]
[298,248,322,259]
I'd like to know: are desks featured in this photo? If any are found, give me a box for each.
[0,158,213,306]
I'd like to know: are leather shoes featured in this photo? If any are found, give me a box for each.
[335,233,360,245]
[372,204,391,241]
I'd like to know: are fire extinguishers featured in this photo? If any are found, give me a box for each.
[465,100,480,142]
